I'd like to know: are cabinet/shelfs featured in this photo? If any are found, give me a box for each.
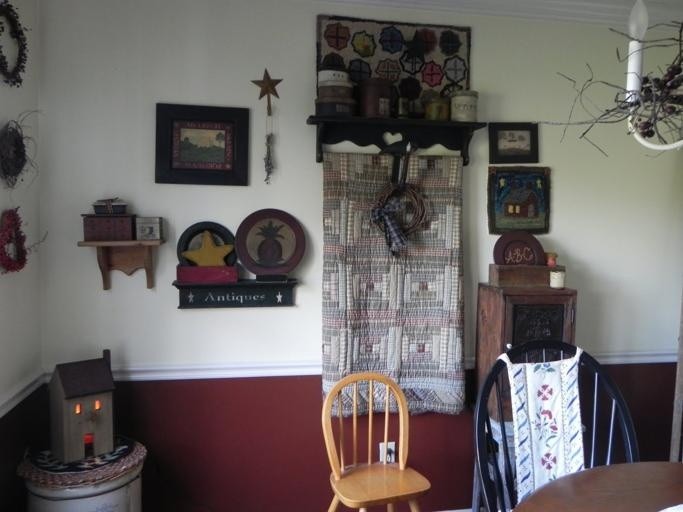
[477,282,577,421]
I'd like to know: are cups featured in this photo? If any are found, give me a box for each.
[550,270,565,288]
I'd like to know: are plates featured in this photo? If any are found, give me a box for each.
[177,210,305,274]
[493,231,546,266]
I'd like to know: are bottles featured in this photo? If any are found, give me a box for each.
[425,89,479,122]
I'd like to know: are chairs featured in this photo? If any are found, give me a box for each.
[476,339,638,512]
[322,373,431,511]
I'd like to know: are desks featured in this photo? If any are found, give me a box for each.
[515,462,682,512]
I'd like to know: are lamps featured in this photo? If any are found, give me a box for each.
[625,1,682,151]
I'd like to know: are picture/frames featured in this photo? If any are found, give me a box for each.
[488,167,550,234]
[488,121,539,163]
[155,102,250,186]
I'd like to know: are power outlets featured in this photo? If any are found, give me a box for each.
[378,442,395,464]
